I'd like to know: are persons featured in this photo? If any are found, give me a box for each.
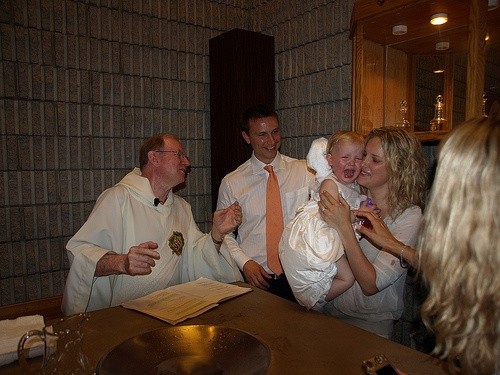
[61,134,244,317]
[278,130,382,311]
[318,125,429,341]
[216,107,317,306]
[356,116,500,375]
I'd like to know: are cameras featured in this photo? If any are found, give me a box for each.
[362,351,400,375]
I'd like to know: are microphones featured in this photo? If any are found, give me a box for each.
[154,198,160,206]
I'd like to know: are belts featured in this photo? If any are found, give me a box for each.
[265,274,286,285]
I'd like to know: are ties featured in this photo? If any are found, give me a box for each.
[263,164,284,278]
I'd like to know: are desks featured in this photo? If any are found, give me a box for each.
[0,281,448,375]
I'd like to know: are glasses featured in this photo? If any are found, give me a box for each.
[154,150,185,158]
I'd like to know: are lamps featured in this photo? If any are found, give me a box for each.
[435,42,449,51]
[430,13,448,25]
[489,0,498,11]
[392,25,407,35]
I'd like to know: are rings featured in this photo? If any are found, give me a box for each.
[321,207,327,213]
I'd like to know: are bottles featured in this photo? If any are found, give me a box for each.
[430,94,449,131]
[396,99,409,133]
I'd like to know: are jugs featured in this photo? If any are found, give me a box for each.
[18,312,97,375]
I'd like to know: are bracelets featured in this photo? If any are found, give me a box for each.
[400,245,411,268]
[211,232,224,244]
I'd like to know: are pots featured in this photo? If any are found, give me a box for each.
[98,325,272,375]
